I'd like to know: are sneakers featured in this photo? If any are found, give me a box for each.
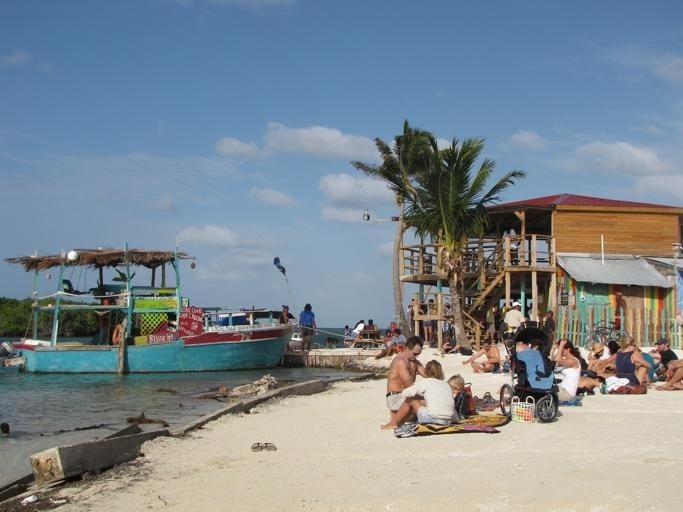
[474,392,498,410]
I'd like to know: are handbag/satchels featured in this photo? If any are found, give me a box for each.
[464,383,476,415]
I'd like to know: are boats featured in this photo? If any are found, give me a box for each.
[0,242,296,374]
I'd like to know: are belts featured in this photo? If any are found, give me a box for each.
[386,391,401,397]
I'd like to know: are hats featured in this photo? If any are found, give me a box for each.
[655,338,667,345]
[512,302,521,307]
[593,343,604,353]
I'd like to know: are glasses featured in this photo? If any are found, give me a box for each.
[412,350,420,355]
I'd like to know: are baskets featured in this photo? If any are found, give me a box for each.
[511,396,535,422]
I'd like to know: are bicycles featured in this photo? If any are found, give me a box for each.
[583,319,629,351]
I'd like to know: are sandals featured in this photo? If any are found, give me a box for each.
[394,423,419,437]
[655,383,683,390]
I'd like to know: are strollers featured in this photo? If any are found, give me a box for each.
[498,318,559,424]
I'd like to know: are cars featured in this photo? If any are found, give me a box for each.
[289,332,303,350]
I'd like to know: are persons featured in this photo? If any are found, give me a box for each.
[376,322,408,359]
[518,336,682,403]
[366,319,375,329]
[381,335,471,429]
[299,303,319,351]
[0,422,10,435]
[468,300,555,373]
[349,320,364,348]
[343,325,350,347]
[502,224,519,266]
[279,305,294,351]
[406,299,473,356]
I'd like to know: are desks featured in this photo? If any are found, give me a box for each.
[358,330,380,349]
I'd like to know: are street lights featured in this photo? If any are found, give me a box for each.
[361,208,405,301]
